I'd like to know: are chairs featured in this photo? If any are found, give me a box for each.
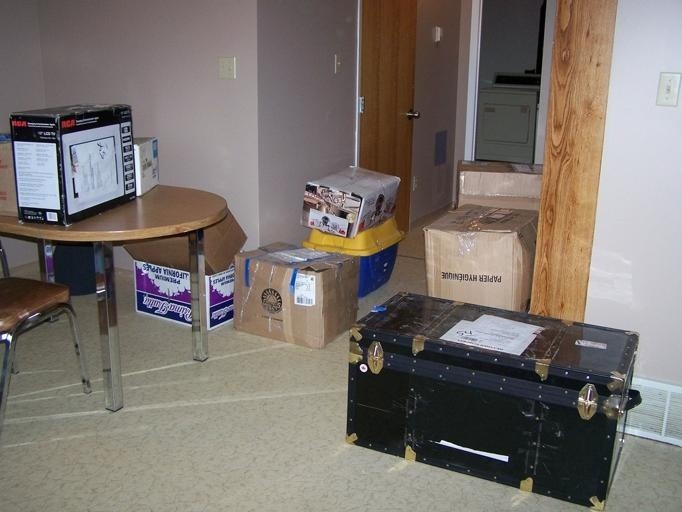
[1,243,91,426]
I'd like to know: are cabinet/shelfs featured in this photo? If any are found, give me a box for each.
[475,73,540,164]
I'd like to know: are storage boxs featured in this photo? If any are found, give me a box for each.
[0,103,158,227]
[134,261,238,331]
[302,166,401,237]
[233,240,359,350]
[425,205,539,313]
[346,291,640,512]
[124,209,247,273]
[456,160,542,210]
[303,217,405,299]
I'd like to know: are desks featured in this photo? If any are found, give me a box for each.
[1,184,227,411]
